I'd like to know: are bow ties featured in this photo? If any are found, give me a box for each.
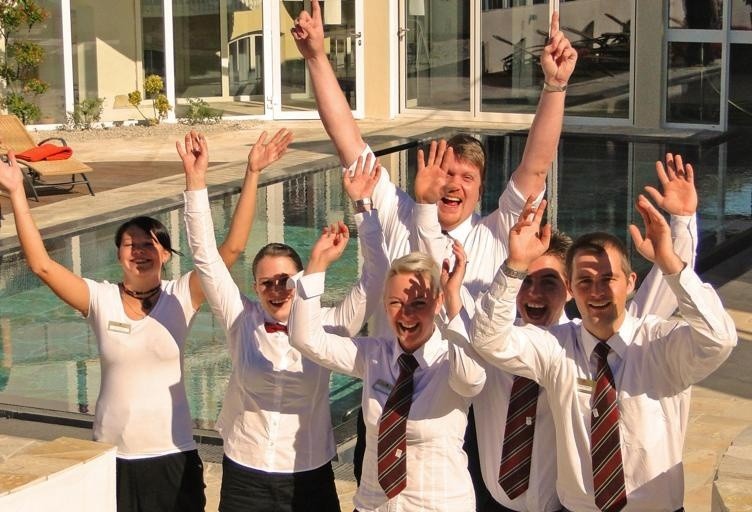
[263,321,289,336]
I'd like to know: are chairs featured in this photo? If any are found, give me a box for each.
[1,112,97,203]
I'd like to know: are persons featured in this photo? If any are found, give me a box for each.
[290,0,580,512]
[176,130,392,512]
[287,221,487,512]
[415,139,698,512]
[0,126,294,512]
[468,194,738,512]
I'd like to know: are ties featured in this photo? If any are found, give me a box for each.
[376,354,422,499]
[589,340,628,512]
[496,374,540,501]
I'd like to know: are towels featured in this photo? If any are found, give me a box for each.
[14,142,73,163]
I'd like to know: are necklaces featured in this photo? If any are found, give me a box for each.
[121,282,161,309]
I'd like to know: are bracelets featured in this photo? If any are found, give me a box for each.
[500,261,528,280]
[543,82,567,92]
[353,197,373,207]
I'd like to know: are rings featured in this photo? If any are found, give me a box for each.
[466,261,469,263]
